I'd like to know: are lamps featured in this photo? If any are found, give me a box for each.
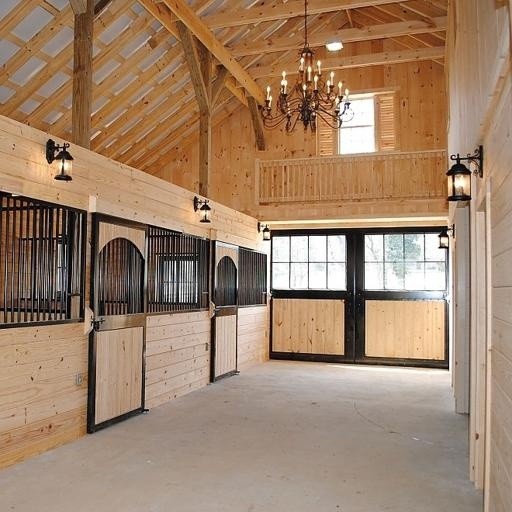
[46,139,74,180]
[261,0,354,133]
[446,145,483,201]
[194,196,211,222]
[258,222,271,240]
[438,224,454,248]
